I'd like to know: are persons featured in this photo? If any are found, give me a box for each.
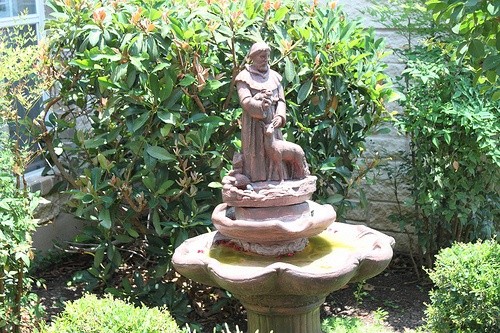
[235,42,293,183]
